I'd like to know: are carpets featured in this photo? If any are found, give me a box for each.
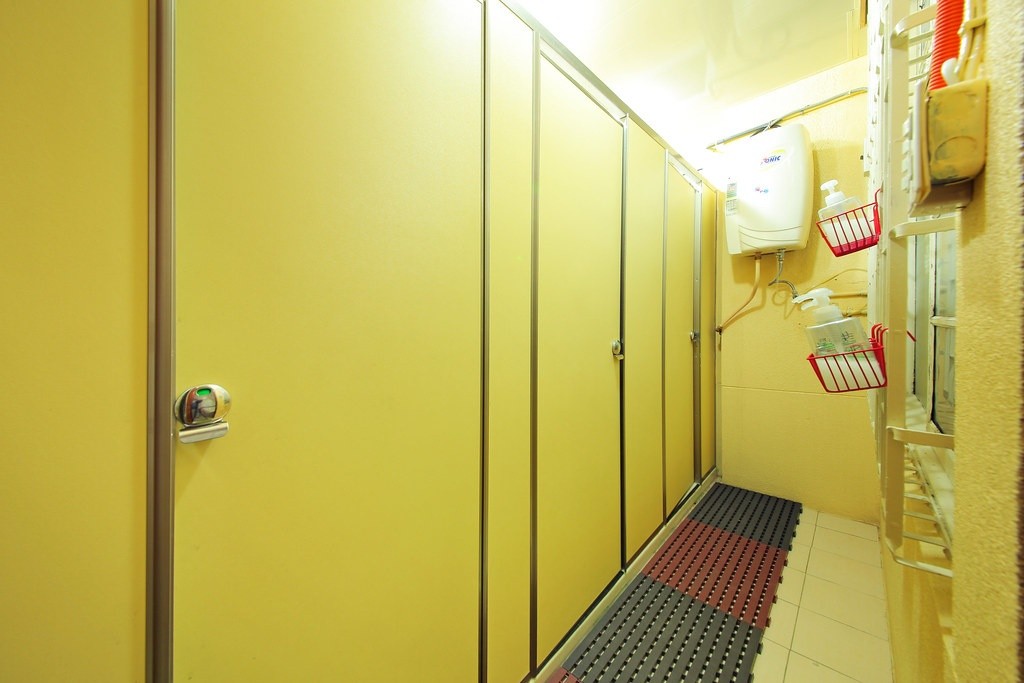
[549,483,804,683]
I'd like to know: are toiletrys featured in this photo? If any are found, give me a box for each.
[817,179,875,248]
[791,288,885,391]
